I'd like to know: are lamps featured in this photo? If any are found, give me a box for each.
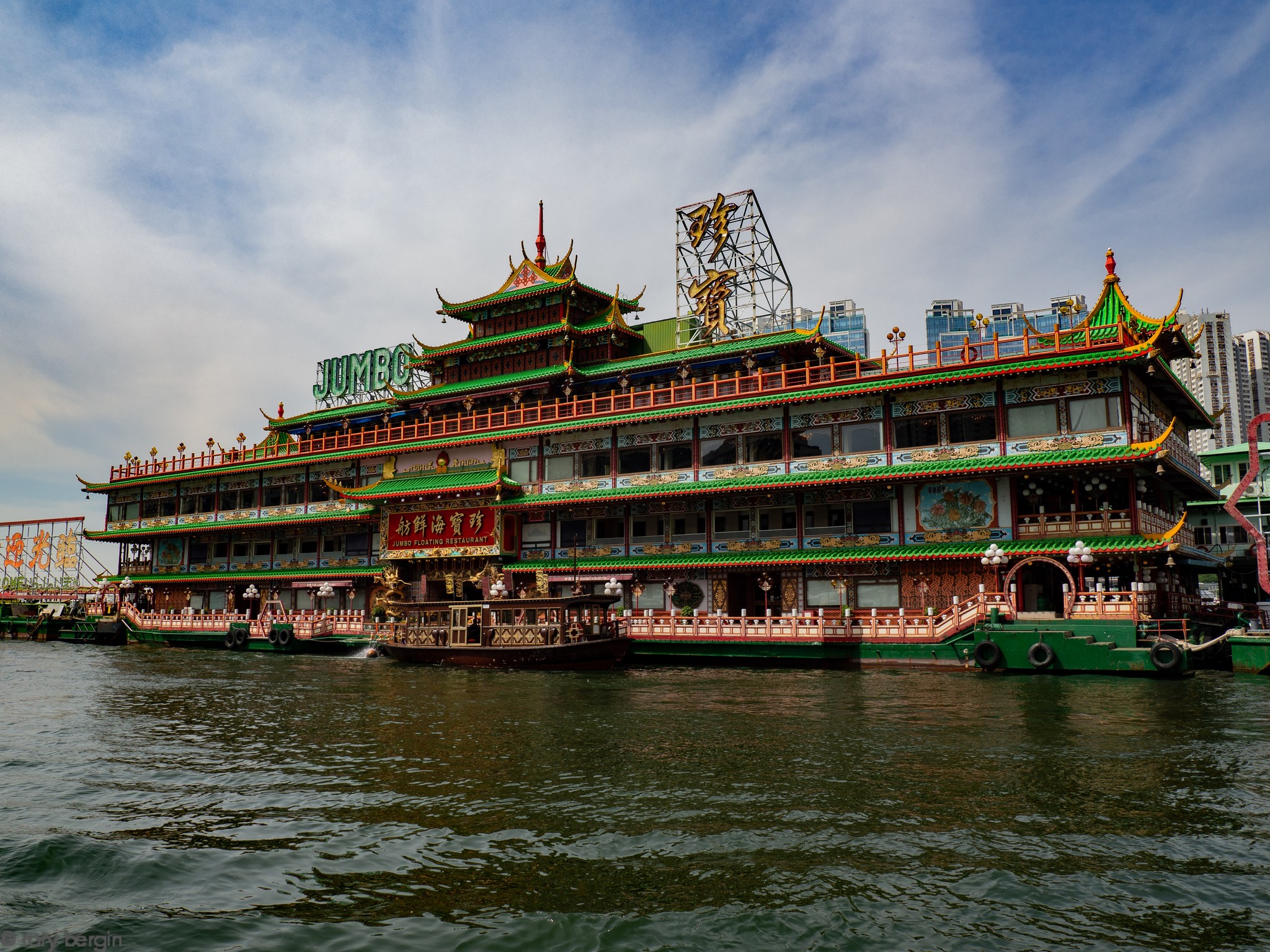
[401,498,408,502]
[384,499,387,504]
[346,503,351,513]
[570,288,577,297]
[418,496,423,501]
[476,489,481,497]
[1146,360,1157,377]
[1189,359,1197,370]
[1209,429,1217,440]
[495,491,502,503]
[85,493,90,500]
[1164,553,1176,568]
[1155,459,1166,476]
[569,375,575,384]
[392,405,397,412]
[610,331,617,340]
[634,312,640,321]
[1220,572,1228,578]
[421,363,426,369]
[436,494,441,499]
[1171,334,1179,345]
[441,314,447,324]
[563,333,569,342]
[1215,501,1222,513]
[456,492,461,498]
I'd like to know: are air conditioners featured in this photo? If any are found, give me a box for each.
[1243,481,1260,496]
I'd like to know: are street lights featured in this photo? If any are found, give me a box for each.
[831,573,850,625]
[96,577,193,614]
[741,350,757,392]
[562,379,574,417]
[887,327,906,372]
[490,580,529,600]
[1060,299,1081,348]
[131,544,151,562]
[1022,477,1107,525]
[758,572,774,617]
[225,583,356,620]
[1138,479,1184,519]
[124,427,279,478]
[812,342,827,382]
[913,572,931,616]
[981,544,1008,593]
[970,313,990,360]
[1144,568,1180,591]
[303,388,523,454]
[1066,541,1094,592]
[677,361,691,401]
[604,577,678,617]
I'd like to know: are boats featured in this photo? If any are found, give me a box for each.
[375,534,636,669]
